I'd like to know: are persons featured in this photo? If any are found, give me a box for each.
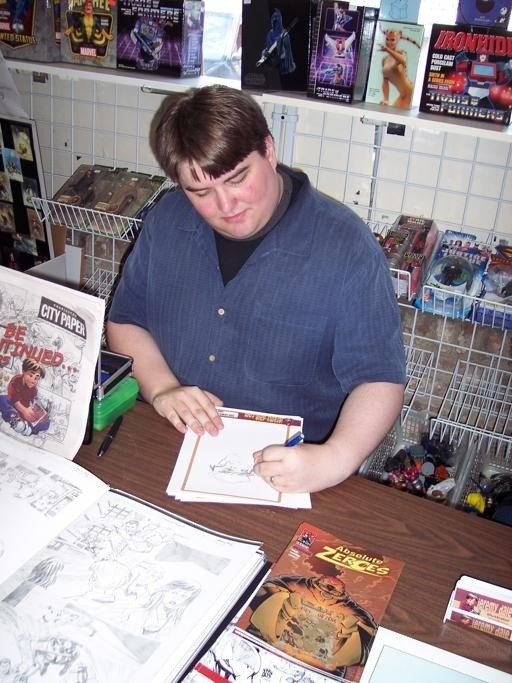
[65,1,113,46]
[106,85,405,488]
[0,359,50,436]
[377,24,420,109]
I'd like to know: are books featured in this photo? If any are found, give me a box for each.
[1,405,512,683]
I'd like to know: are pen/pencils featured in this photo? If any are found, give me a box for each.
[97,416,123,457]
[249,432,305,476]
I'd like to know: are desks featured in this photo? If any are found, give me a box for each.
[74,399,512,681]
